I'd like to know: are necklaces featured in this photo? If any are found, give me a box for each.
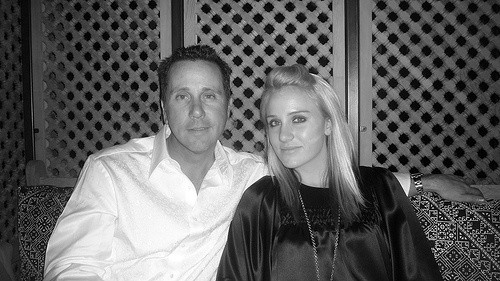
[297,188,341,280]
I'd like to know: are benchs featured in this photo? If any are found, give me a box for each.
[15,160,500,280]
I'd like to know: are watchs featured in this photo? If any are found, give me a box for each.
[410,173,425,195]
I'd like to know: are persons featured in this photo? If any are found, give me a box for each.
[36,45,485,281]
[217,66,445,281]
